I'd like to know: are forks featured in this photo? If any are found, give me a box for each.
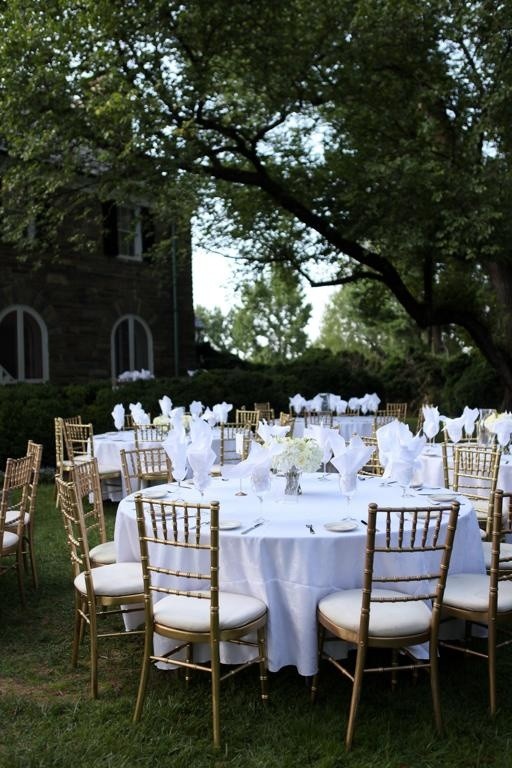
[306,525,315,534]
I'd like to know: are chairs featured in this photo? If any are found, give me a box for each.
[430,489,511,717]
[0,452,35,609]
[54,403,511,540]
[0,440,43,590]
[133,495,268,749]
[67,458,117,644]
[309,502,460,753]
[54,474,144,698]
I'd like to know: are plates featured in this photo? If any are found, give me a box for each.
[218,520,240,529]
[433,497,456,501]
[418,514,437,519]
[150,495,165,498]
[324,522,356,531]
[148,510,173,515]
[411,480,423,486]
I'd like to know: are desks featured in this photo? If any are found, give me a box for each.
[119,471,488,677]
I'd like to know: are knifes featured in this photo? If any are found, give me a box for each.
[361,520,378,531]
[241,522,262,534]
